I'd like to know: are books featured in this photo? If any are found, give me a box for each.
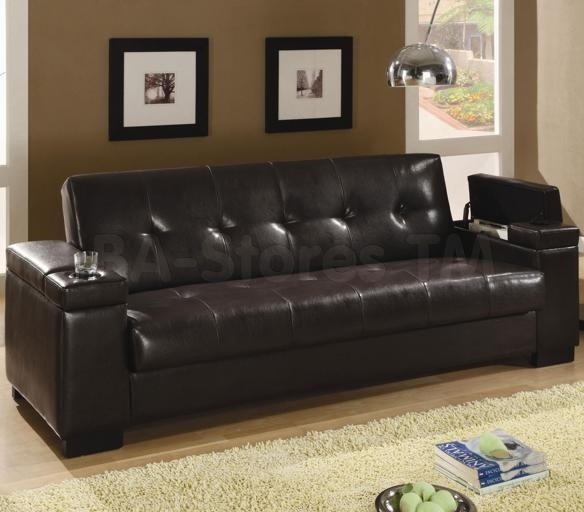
[433,428,549,495]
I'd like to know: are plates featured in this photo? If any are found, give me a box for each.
[374,483,476,511]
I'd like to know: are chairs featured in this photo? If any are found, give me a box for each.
[467,171,564,225]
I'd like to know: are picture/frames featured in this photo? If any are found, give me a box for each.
[107,36,211,143]
[263,36,355,131]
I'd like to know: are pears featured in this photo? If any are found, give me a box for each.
[398,482,457,511]
[475,430,507,455]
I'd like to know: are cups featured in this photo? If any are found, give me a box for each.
[73,250,98,276]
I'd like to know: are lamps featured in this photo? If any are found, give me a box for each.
[386,2,460,88]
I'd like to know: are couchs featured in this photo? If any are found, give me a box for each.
[4,153,579,457]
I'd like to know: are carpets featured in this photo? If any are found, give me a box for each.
[0,375,584,511]
